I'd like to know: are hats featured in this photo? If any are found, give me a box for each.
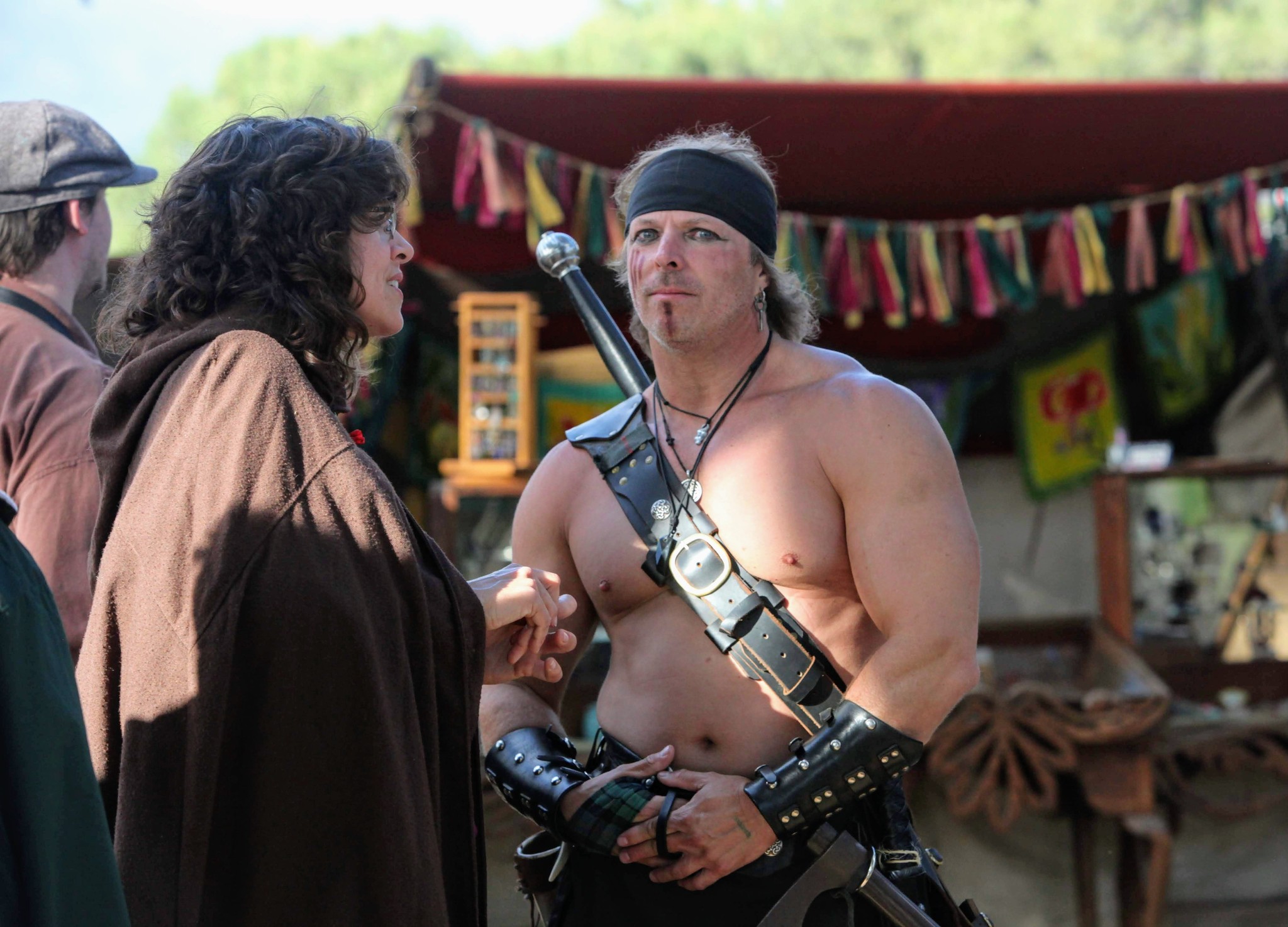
[0,99,157,215]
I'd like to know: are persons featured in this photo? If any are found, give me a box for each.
[1,110,580,927]
[476,127,983,927]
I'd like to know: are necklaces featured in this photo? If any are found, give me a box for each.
[653,321,774,537]
